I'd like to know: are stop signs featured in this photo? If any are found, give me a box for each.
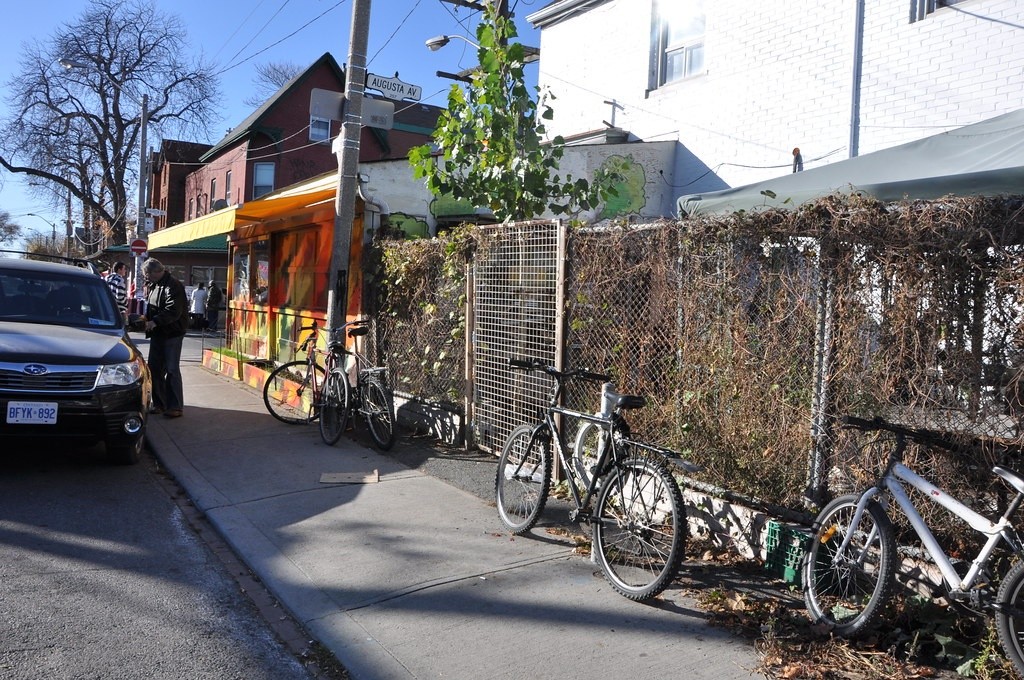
[129,238,151,258]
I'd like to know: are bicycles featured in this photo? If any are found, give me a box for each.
[494,361,691,604]
[801,414,1024,675]
[261,319,397,450]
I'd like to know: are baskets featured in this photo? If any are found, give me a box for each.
[766,521,844,593]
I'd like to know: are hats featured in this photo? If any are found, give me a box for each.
[198,283,204,288]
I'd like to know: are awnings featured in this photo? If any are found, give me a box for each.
[82,238,129,261]
[147,190,336,254]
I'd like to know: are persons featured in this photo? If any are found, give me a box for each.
[104,260,128,334]
[190,279,222,333]
[136,257,189,419]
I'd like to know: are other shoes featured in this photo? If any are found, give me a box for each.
[165,409,183,419]
[149,406,162,414]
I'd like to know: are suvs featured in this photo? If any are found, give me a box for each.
[0,257,151,463]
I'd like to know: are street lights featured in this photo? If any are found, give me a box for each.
[26,214,57,263]
[29,182,72,267]
[28,227,48,251]
